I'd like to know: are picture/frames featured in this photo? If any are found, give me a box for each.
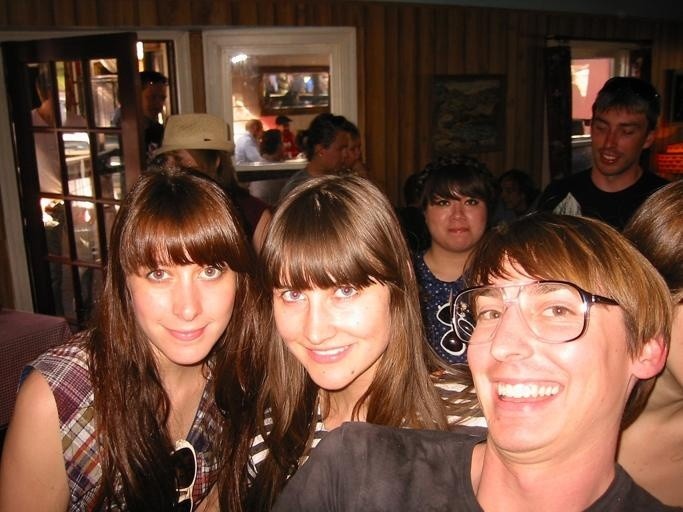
[256,65,331,117]
[425,72,510,159]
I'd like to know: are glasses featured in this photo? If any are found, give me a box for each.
[170,439,197,512]
[452,281,618,344]
[142,80,169,91]
[437,304,466,356]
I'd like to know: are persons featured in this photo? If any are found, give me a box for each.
[0,68,682,510]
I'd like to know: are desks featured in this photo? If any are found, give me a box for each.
[1,310,76,454]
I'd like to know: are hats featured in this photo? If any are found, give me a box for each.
[276,116,292,124]
[152,114,235,159]
